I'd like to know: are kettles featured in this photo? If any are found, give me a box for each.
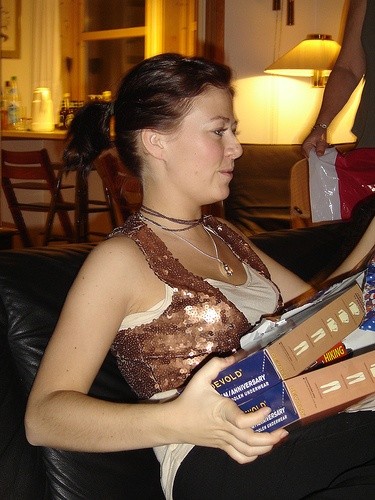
[31,87,56,132]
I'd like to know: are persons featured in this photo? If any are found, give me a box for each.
[24,53,375,500]
[301,0,375,159]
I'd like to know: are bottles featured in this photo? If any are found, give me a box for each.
[102,90,115,136]
[0,77,22,129]
[59,98,84,131]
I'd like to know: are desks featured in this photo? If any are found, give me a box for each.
[1,130,118,233]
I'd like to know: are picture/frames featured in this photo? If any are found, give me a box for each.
[1,0,22,58]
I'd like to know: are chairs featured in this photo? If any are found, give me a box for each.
[0,143,224,249]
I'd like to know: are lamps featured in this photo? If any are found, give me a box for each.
[264,33,341,87]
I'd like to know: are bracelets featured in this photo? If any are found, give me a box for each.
[312,122,327,129]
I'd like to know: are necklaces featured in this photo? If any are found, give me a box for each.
[135,204,234,278]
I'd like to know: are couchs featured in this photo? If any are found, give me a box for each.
[0,195,375,500]
